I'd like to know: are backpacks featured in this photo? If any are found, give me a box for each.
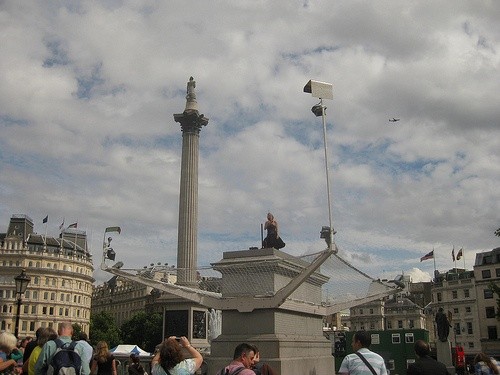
[251,362,264,375]
[47,338,82,375]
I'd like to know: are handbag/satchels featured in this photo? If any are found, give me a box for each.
[274,238,285,249]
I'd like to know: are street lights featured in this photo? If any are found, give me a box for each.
[15,268,32,338]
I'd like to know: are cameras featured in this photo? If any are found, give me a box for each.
[175,337,181,343]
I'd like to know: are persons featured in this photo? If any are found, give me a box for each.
[338,330,387,375]
[248,345,276,375]
[435,308,452,343]
[151,335,203,375]
[77,332,93,375]
[263,213,286,250]
[90,341,117,375]
[475,353,499,375]
[128,357,148,375]
[35,322,91,375]
[407,340,449,375]
[215,343,256,375]
[0,327,58,375]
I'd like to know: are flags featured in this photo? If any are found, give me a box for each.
[43,216,47,223]
[68,223,77,228]
[456,248,463,260]
[420,251,434,262]
[451,248,455,262]
